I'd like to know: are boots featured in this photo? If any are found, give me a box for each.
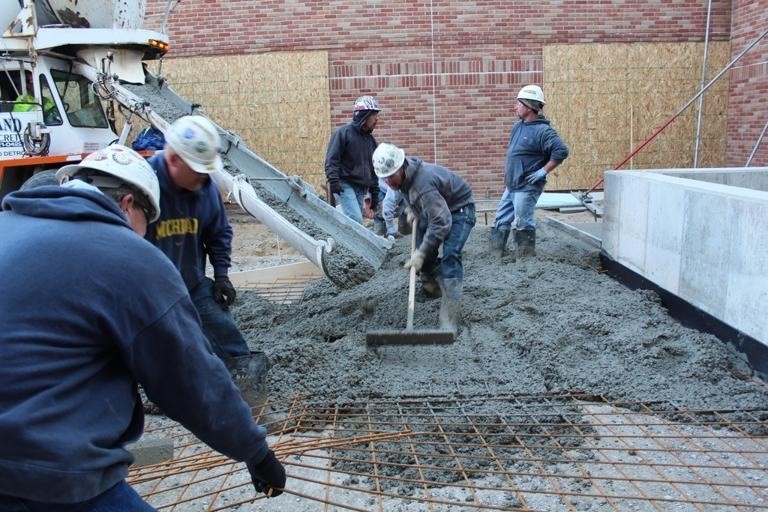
[371,215,410,242]
[476,225,510,261]
[497,227,536,261]
[413,267,442,304]
[439,274,463,342]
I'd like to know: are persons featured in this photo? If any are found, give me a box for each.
[0,144,285,508]
[138,116,249,373]
[489,85,569,262]
[368,179,411,237]
[325,93,379,221]
[371,142,476,339]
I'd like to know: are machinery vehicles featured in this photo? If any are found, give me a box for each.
[1,0,396,291]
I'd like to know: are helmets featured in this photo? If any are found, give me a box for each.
[515,85,548,108]
[354,96,382,112]
[54,143,161,226]
[370,141,407,178]
[162,115,225,176]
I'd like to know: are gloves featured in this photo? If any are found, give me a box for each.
[404,249,425,273]
[325,181,346,198]
[523,166,548,185]
[246,451,288,497]
[369,191,380,212]
[207,275,237,309]
